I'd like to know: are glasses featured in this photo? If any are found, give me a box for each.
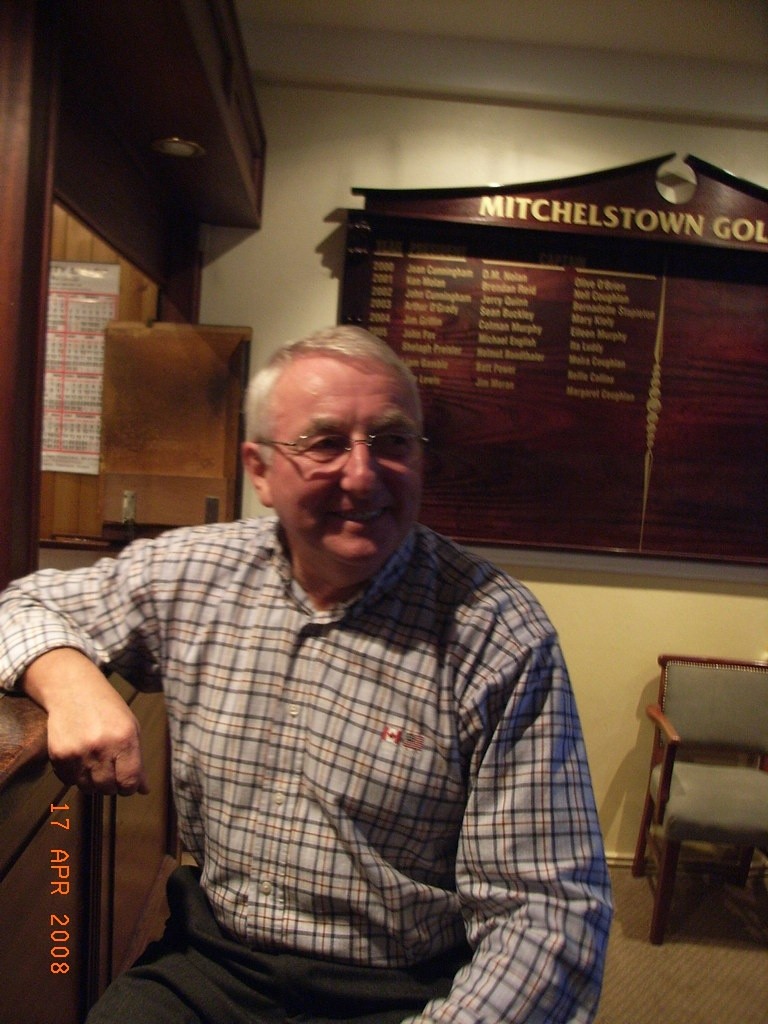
[258,430,431,468]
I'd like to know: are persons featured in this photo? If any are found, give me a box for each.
[0,328,611,1024]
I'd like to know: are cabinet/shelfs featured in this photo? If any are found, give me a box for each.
[0,670,181,1024]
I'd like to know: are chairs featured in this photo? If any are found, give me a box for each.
[632,654,768,945]
